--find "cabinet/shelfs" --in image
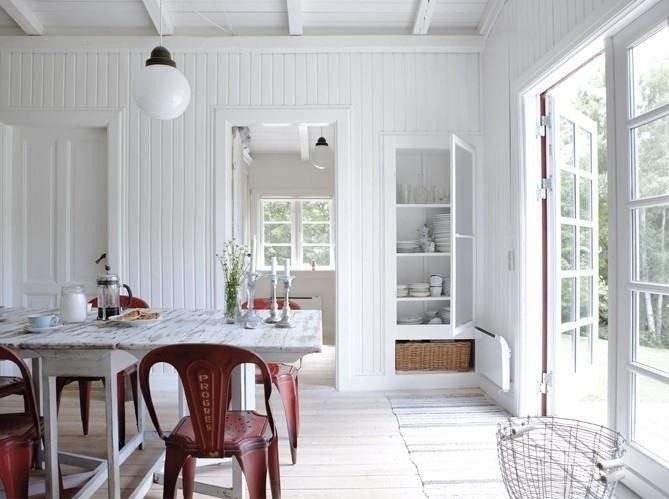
[383,135,511,395]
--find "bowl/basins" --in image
[440,305,451,323]
[424,309,440,324]
[429,275,443,297]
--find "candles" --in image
[250,235,290,277]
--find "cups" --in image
[86,303,92,313]
[398,182,412,204]
[432,184,450,204]
[27,313,59,328]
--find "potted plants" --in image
[214,237,251,322]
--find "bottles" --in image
[59,284,87,323]
[311,258,316,270]
[413,170,428,204]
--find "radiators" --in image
[475,326,511,394]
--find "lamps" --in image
[310,127,331,169]
[134,0,190,122]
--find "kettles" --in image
[95,272,132,322]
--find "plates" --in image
[428,317,442,324]
[108,313,164,327]
[431,213,451,252]
[25,326,58,334]
[397,284,408,297]
[408,282,431,297]
[397,315,423,324]
[396,240,418,253]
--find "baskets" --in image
[496,416,628,499]
[395,341,472,371]
